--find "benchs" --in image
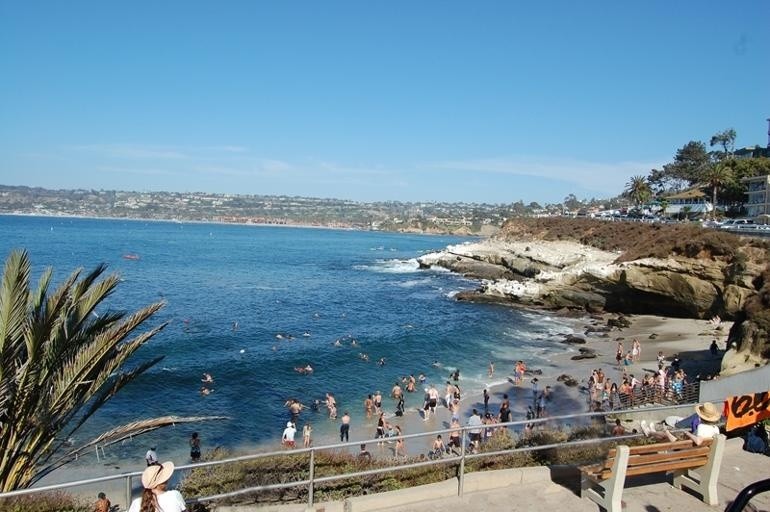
[576,432,727,512]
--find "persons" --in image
[710,341,719,358]
[127,460,188,512]
[612,419,624,436]
[588,340,689,423]
[189,433,201,462]
[640,402,722,451]
[467,360,553,454]
[695,367,721,382]
[201,319,462,463]
[93,491,112,512]
[144,443,158,466]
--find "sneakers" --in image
[640,420,651,438]
[650,422,656,434]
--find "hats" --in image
[141,461,175,489]
[151,444,158,448]
[695,401,721,423]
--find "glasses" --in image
[151,462,163,481]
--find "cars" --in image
[593,208,756,229]
